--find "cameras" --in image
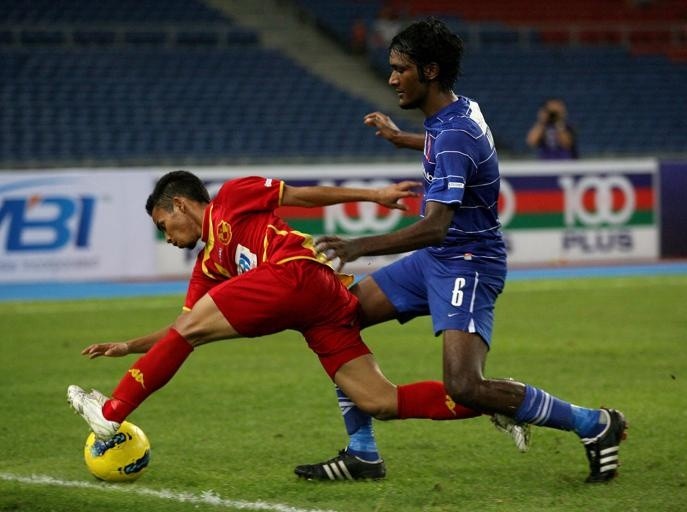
[547,111,558,127]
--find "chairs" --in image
[1,1,686,169]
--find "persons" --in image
[63,168,534,456]
[527,97,579,160]
[293,17,627,482]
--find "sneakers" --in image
[294,444,386,482]
[66,384,120,441]
[579,406,629,483]
[493,376,531,454]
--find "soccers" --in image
[84,420,151,483]
